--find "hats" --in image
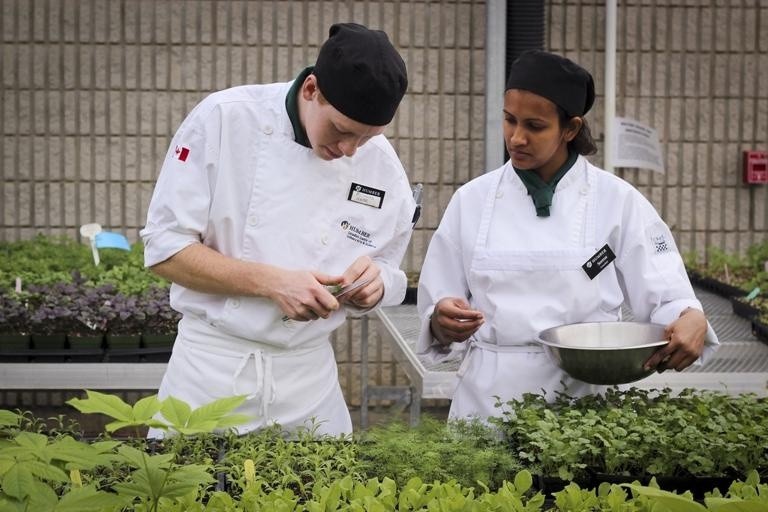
[502,48,596,117]
[310,23,408,127]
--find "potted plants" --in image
[684,238,768,345]
[0,266,184,364]
[57,433,500,507]
[514,388,768,502]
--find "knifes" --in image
[279,278,370,323]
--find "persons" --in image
[418,50,721,447]
[136,23,419,439]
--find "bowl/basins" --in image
[533,320,677,386]
